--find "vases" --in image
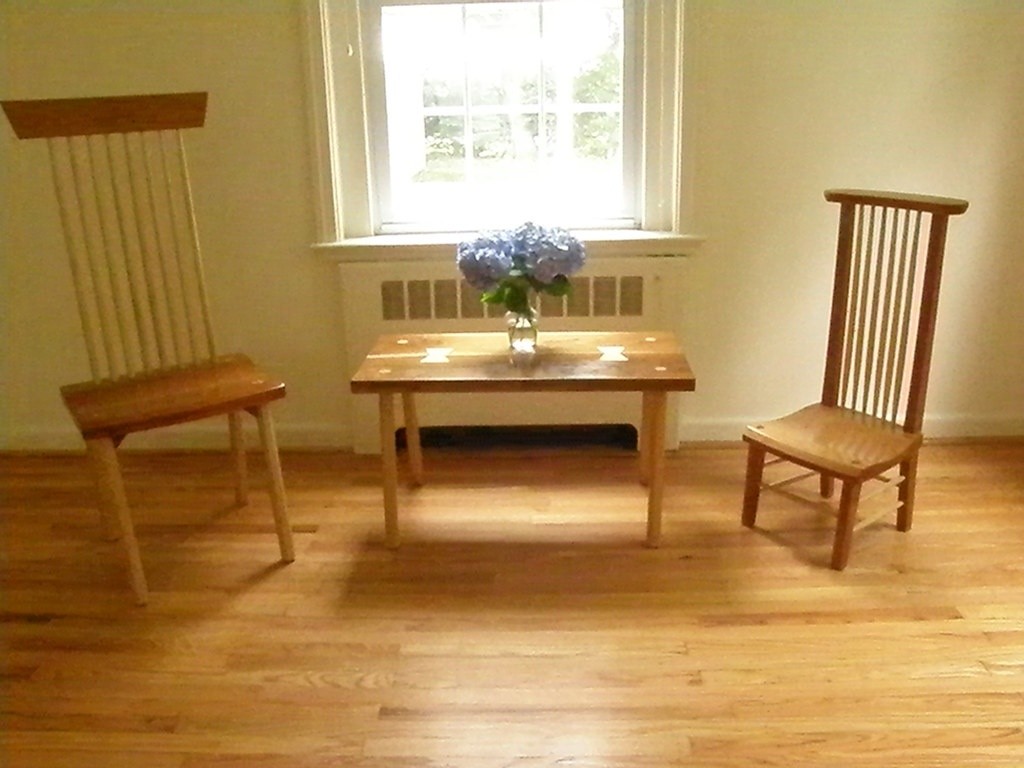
[504,310,537,351]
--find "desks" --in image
[350,332,697,549]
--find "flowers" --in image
[456,221,586,344]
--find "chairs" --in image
[0,92,295,607]
[742,190,969,571]
